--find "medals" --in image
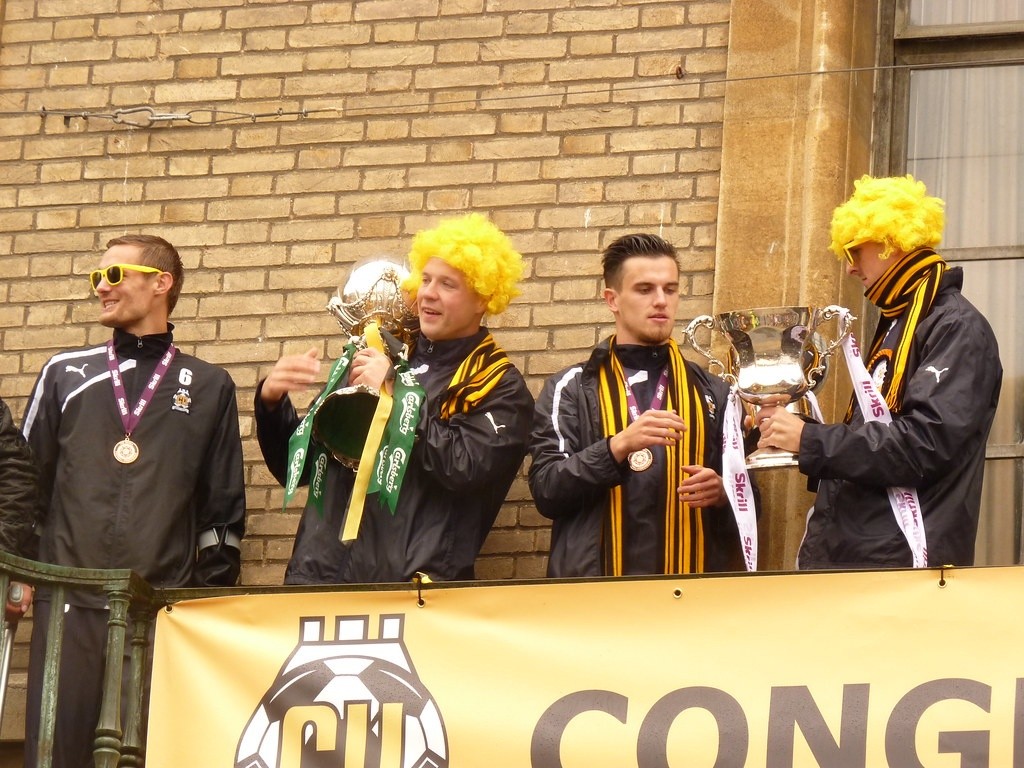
[623,444,654,471]
[111,431,141,464]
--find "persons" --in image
[527,234,761,578]
[253,211,536,585]
[755,172,1004,569]
[1,233,245,767]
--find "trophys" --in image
[322,252,419,405]
[681,303,859,470]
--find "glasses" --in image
[842,237,871,267]
[89,263,162,290]
